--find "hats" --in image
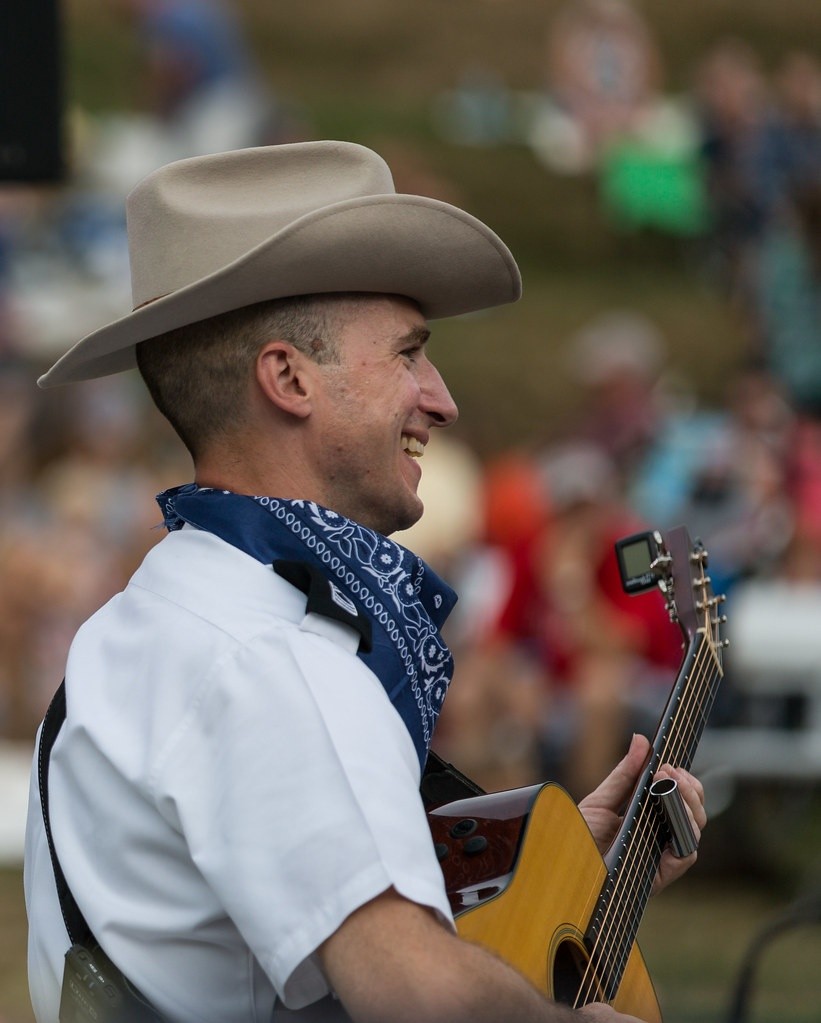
[29,140,523,383]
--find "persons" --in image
[22,140,706,1022]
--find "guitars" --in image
[415,523,736,1022]
